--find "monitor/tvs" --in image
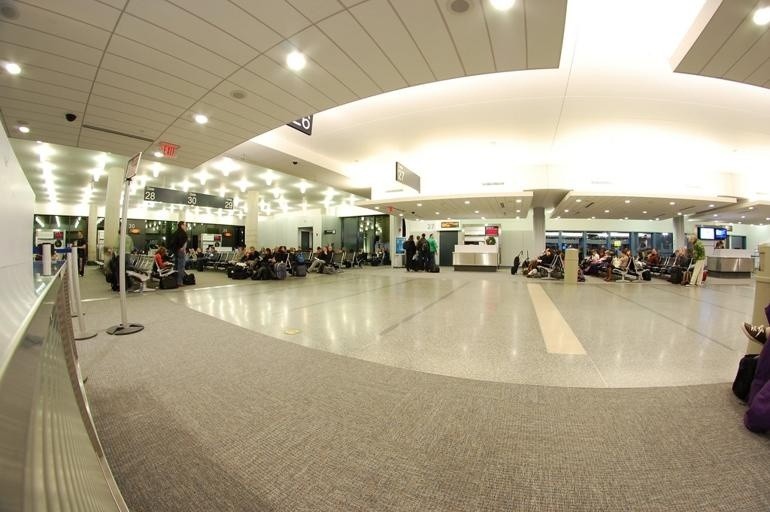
[484,226,498,236]
[715,228,728,240]
[699,227,715,240]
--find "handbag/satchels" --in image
[733,354,760,403]
[613,257,622,269]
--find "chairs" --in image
[95,250,366,292]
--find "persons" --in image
[742,302,769,344]
[524,233,705,288]
[405,233,438,272]
[170,221,188,286]
[714,240,724,248]
[104,228,135,292]
[227,245,304,277]
[306,244,335,273]
[186,244,219,271]
[129,247,170,269]
[376,244,388,266]
[76,230,88,278]
[146,240,165,249]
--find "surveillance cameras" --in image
[65,114,77,122]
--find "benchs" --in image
[533,255,697,284]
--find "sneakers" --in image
[741,321,767,345]
